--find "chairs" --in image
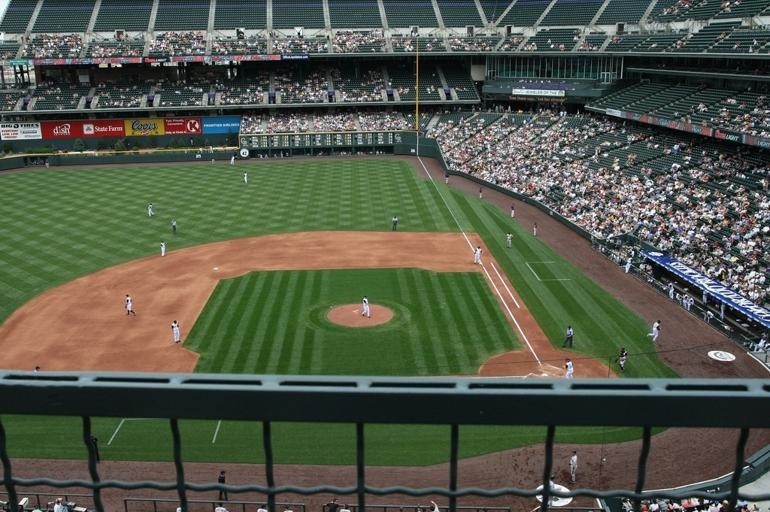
[620,495,762,512]
[0,0,436,157]
[427,3,768,313]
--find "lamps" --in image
[620,495,762,512]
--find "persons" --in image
[244,171,247,183]
[33,498,759,512]
[2,29,436,169]
[533,222,537,236]
[562,325,574,348]
[647,320,662,341]
[160,241,167,256]
[171,218,177,231]
[218,471,228,501]
[474,246,483,264]
[171,320,181,343]
[148,203,154,216]
[362,296,371,317]
[437,0,770,366]
[90,435,100,462]
[565,358,574,379]
[547,474,555,509]
[511,203,515,217]
[569,450,578,482]
[615,348,627,370]
[392,216,398,231]
[506,232,513,248]
[34,366,40,372]
[445,172,449,184]
[479,187,482,198]
[125,294,136,316]
